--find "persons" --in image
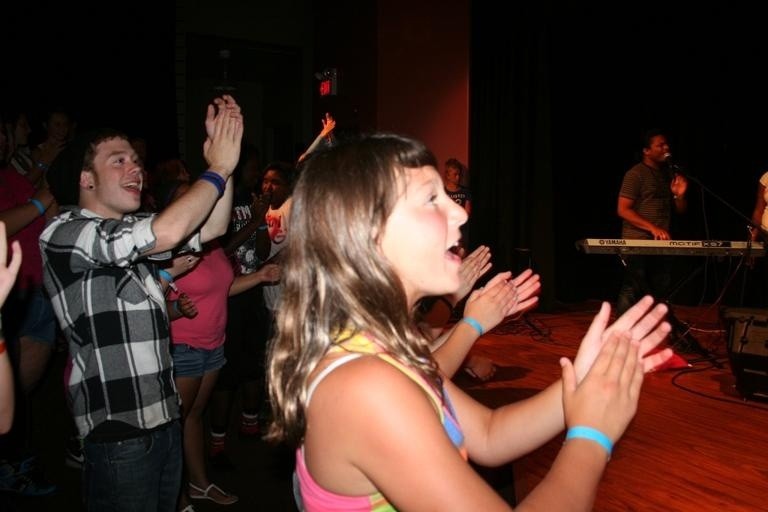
[615,132,688,313]
[0,96,541,511]
[749,172,768,309]
[269,131,673,510]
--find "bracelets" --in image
[566,426,612,461]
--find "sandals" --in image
[180,505,194,512]
[189,482,238,504]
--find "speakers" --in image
[719,306,767,402]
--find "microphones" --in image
[663,152,676,183]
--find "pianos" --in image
[572,238,764,256]
